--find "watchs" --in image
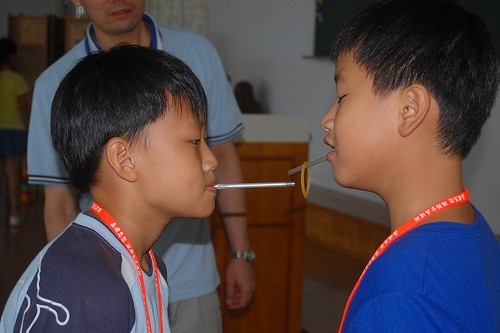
[231,250,257,262]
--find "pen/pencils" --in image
[213,181,296,189]
[287,156,327,175]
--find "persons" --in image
[27,0,256,333]
[321,0,500,333]
[0,44,218,333]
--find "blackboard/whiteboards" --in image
[315,0,500,62]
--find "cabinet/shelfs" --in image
[207,143,309,333]
